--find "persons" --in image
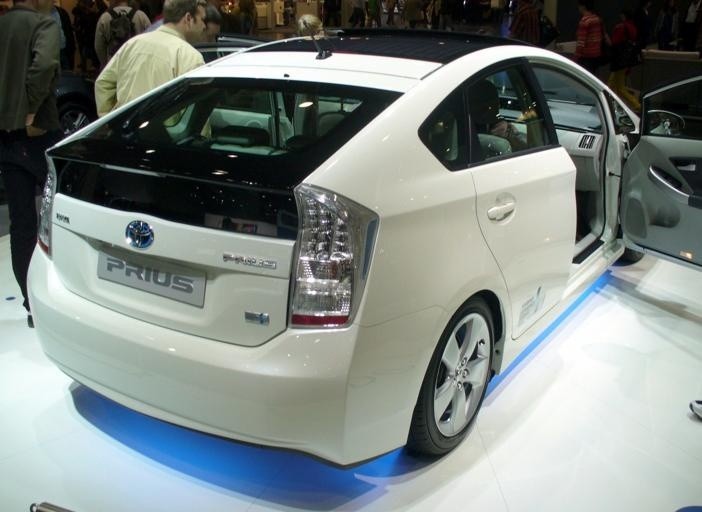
[321,1,700,106]
[1,1,66,331]
[469,80,528,147]
[63,1,258,135]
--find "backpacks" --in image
[104,6,138,57]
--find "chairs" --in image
[479,133,513,157]
[209,113,351,157]
